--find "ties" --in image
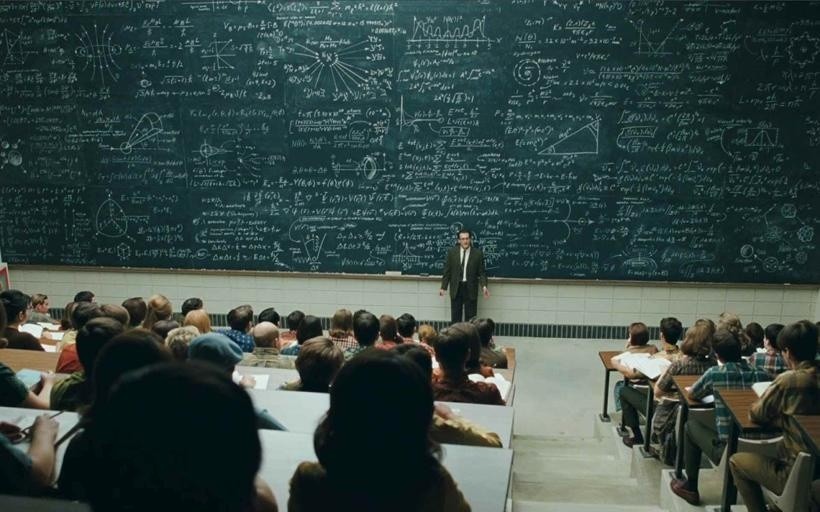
[461,249,466,281]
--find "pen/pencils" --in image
[26,410,65,430]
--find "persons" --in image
[438,229,490,324]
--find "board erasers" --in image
[384,271,402,276]
[420,273,430,276]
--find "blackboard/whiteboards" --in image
[0,0,820,289]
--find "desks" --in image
[1,321,88,478]
[230,327,516,449]
[254,428,517,512]
[597,350,820,511]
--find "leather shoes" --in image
[670,477,700,505]
[621,436,634,449]
